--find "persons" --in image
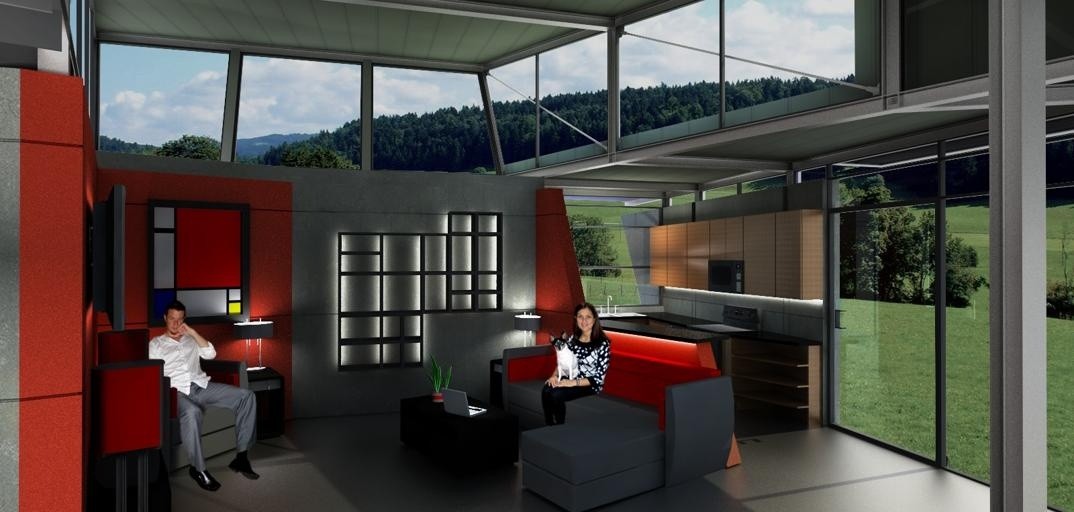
[542,303,610,426]
[148,300,259,491]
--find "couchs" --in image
[503,343,738,511]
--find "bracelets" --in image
[576,379,579,386]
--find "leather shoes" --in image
[189,464,221,491]
[228,457,260,480]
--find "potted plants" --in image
[424,355,454,402]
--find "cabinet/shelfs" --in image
[648,208,824,305]
[733,338,823,430]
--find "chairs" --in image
[152,359,250,477]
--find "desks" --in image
[247,370,285,441]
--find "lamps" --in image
[512,312,544,348]
[234,320,274,374]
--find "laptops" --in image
[441,386,489,418]
